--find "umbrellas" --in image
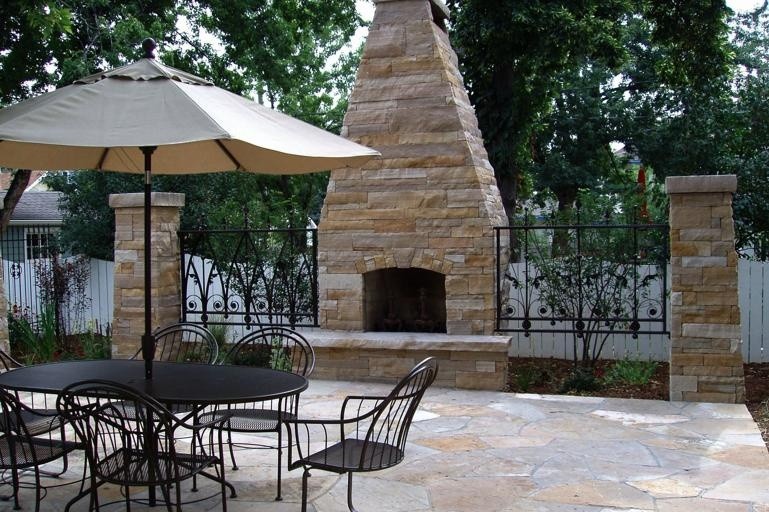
[1,38,383,507]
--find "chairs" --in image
[191,327,317,499]
[57,380,235,512]
[282,355,437,510]
[1,358,310,498]
[101,321,219,491]
[0,350,98,511]
